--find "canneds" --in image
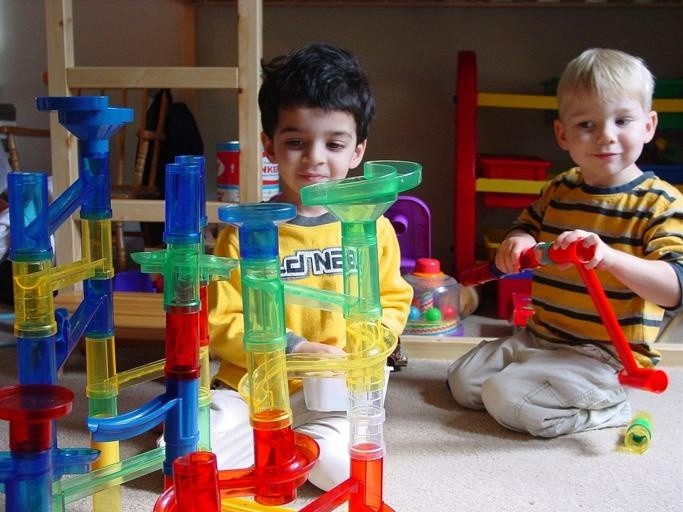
[214,139,281,204]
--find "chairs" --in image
[0,64,174,271]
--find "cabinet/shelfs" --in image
[44,0,264,356]
[455,48,682,293]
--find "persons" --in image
[155,42,415,494]
[444,47,683,442]
